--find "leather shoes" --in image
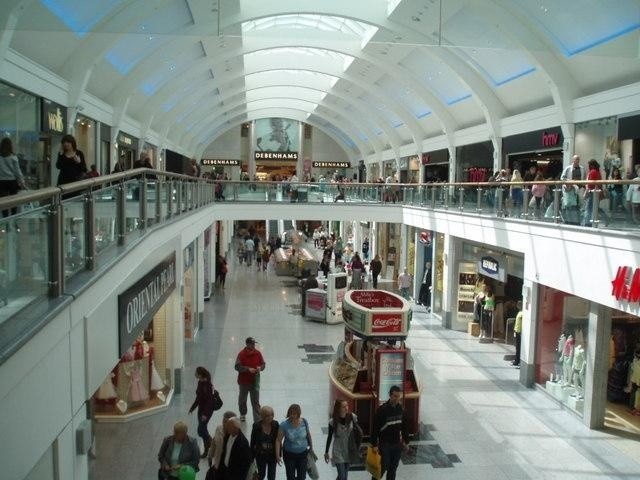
[206,437,212,447]
[202,448,209,457]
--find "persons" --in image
[551,329,588,399]
[1,134,200,235]
[202,169,402,204]
[417,262,431,304]
[471,276,496,341]
[512,301,523,368]
[219,222,411,300]
[157,336,413,480]
[408,155,640,229]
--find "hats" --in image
[246,338,257,344]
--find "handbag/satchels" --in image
[247,462,259,480]
[349,431,363,464]
[158,461,169,480]
[211,386,224,410]
[365,446,381,479]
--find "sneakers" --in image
[240,415,245,421]
